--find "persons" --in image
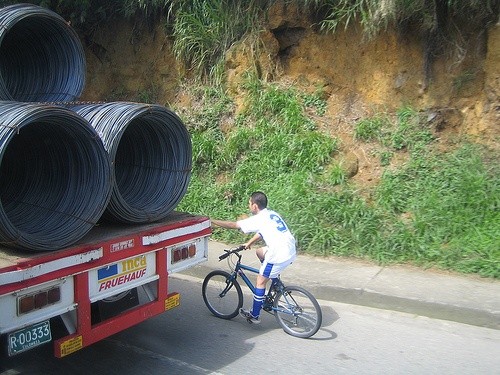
[210,191,298,325]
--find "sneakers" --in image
[239,308,261,324]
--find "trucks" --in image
[0,215,210,356]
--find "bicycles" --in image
[201,245,323,338]
